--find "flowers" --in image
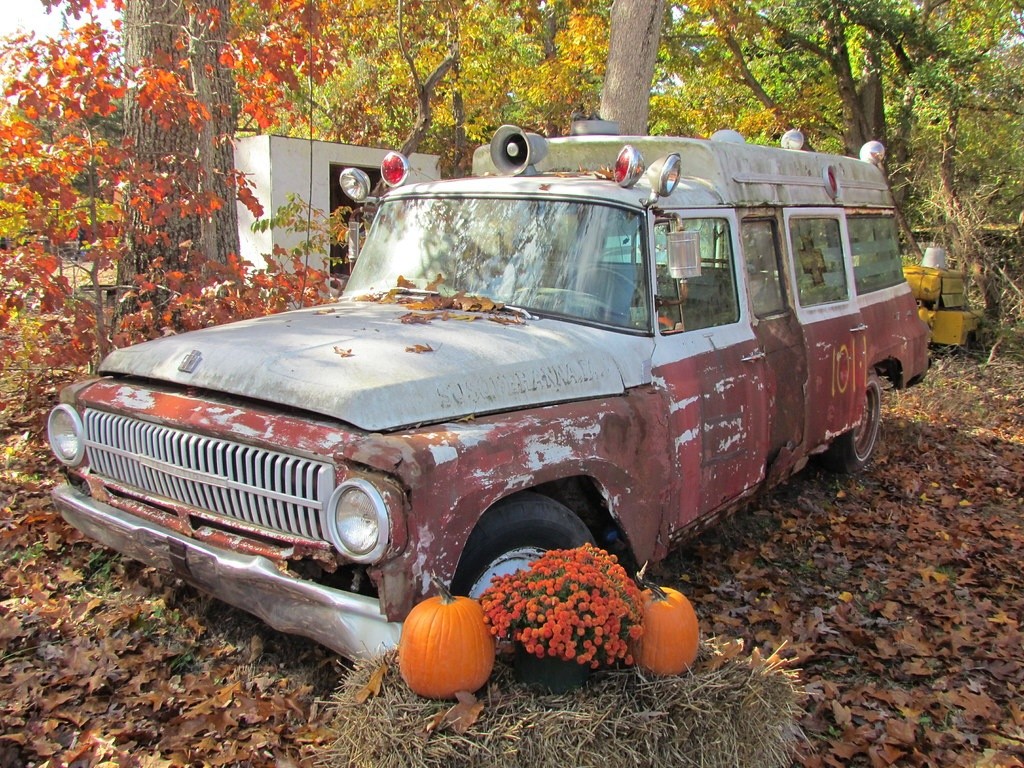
[477,541,647,671]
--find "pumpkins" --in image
[397,576,495,700]
[633,560,699,674]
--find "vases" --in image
[507,630,598,692]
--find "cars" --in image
[49,117,930,664]
